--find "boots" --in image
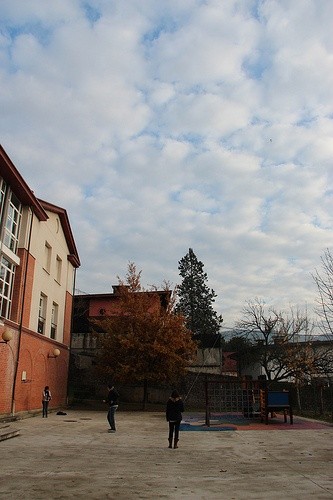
[168,437,172,448]
[174,438,179,449]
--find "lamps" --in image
[47,349,60,358]
[0,330,13,344]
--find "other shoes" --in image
[108,429,116,432]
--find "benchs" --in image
[267,391,293,424]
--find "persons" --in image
[165,392,185,448]
[103,383,119,431]
[42,386,51,418]
